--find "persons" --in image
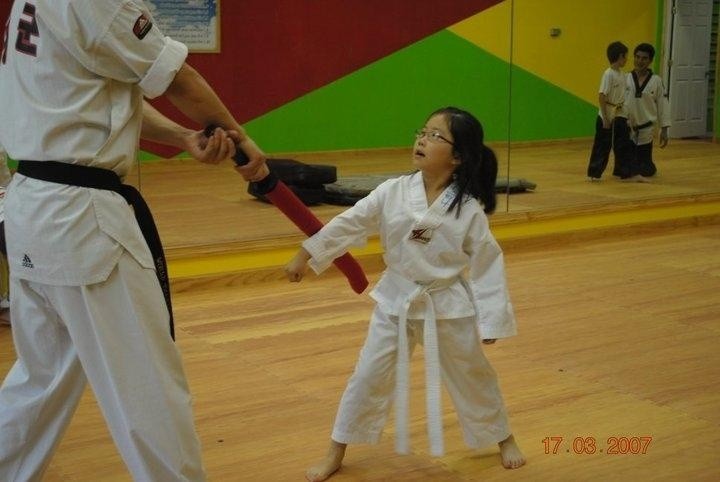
[0,138,13,327]
[627,43,671,177]
[588,41,650,183]
[286,106,527,481]
[0,0,269,480]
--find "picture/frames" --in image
[143,0,222,54]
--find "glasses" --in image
[415,127,453,146]
